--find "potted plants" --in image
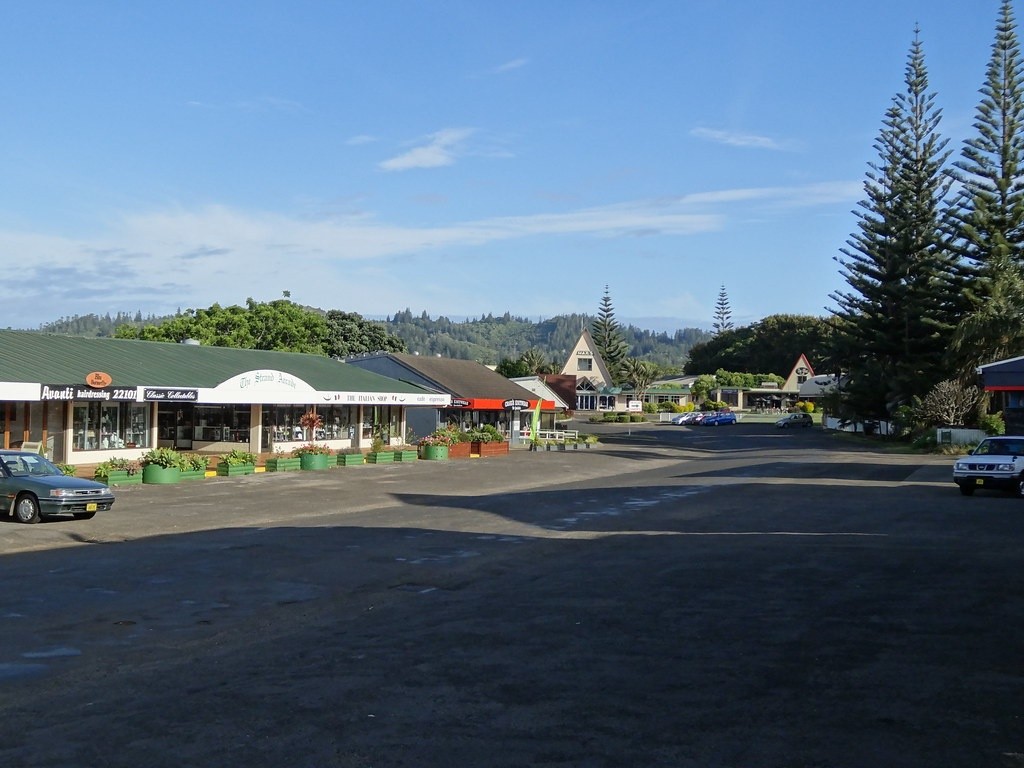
[433,422,509,459]
[93,440,419,488]
[530,436,600,453]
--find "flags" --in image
[529,399,542,439]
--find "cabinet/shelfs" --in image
[72,401,151,451]
[176,404,250,447]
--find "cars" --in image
[776,413,814,428]
[671,411,737,427]
[953,436,1024,499]
[0,450,116,524]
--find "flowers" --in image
[302,444,334,455]
[420,434,450,447]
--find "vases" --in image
[424,446,447,460]
[300,454,328,470]
